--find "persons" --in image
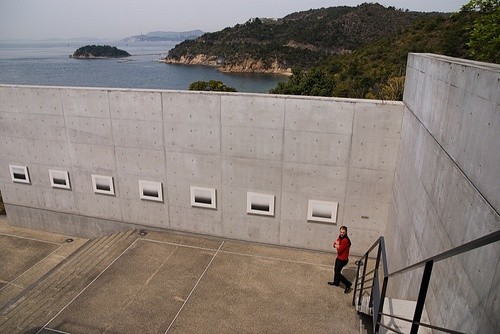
[327,226,353,294]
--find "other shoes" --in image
[328,282,340,286]
[344,283,352,293]
